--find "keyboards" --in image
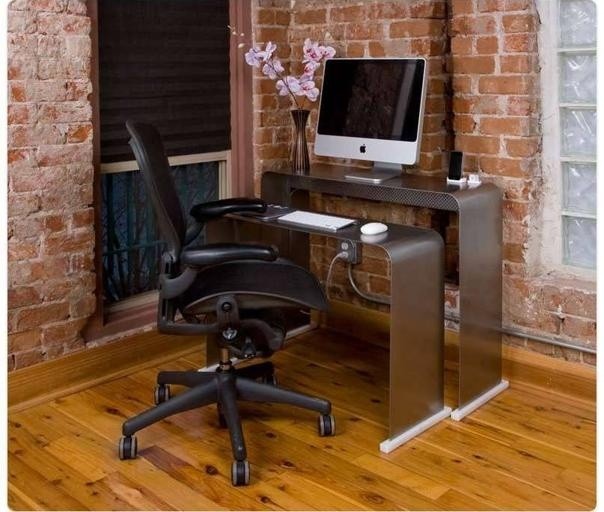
[277,210,356,232]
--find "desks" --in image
[258,165,508,424]
[213,202,455,453]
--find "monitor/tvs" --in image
[314,58,427,182]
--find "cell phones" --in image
[449,151,463,180]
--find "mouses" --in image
[360,223,388,233]
[361,232,389,244]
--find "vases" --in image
[290,111,309,173]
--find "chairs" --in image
[117,118,335,485]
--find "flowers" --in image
[227,23,337,110]
